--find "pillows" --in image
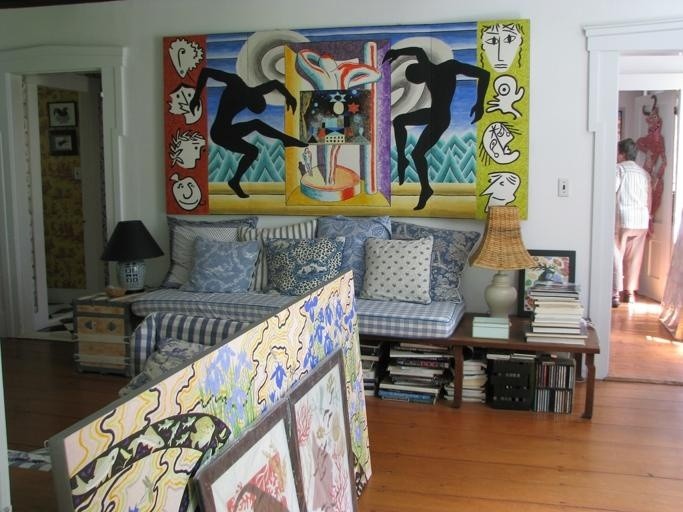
[168,216,256,291]
[390,223,478,304]
[123,340,215,394]
[266,237,344,299]
[239,218,317,292]
[364,237,433,304]
[318,216,392,297]
[179,239,259,296]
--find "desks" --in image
[447,314,598,421]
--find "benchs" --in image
[132,285,467,339]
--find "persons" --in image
[612,138,653,308]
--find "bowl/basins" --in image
[105,288,127,296]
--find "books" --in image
[377,342,455,405]
[486,352,510,361]
[462,359,488,404]
[360,341,377,396]
[525,284,589,346]
[535,353,575,413]
[510,351,536,359]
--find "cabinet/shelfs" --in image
[72,290,150,377]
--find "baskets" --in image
[488,358,536,411]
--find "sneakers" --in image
[624,294,636,303]
[612,295,621,307]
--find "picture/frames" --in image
[518,250,577,317]
[189,398,311,512]
[48,131,78,156]
[47,102,76,126]
[287,344,358,512]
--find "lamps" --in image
[100,220,164,294]
[469,207,540,326]
